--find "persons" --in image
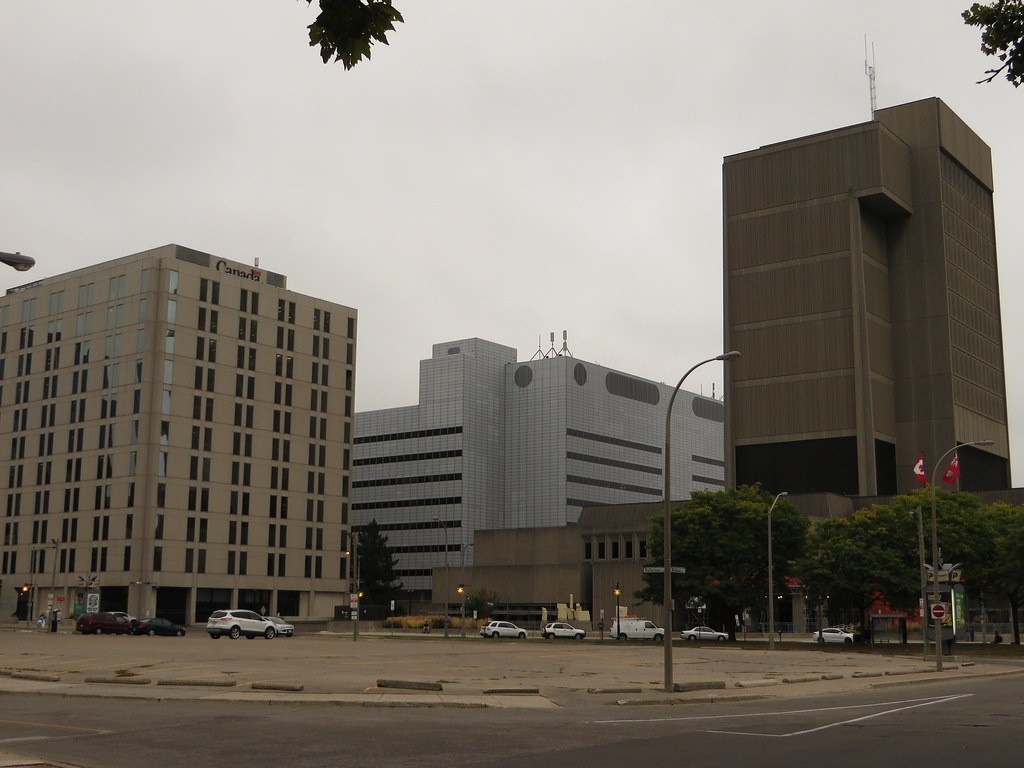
[990,630,998,644]
[422,621,430,633]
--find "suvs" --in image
[541,622,586,641]
[206,609,295,640]
[75,611,137,635]
[480,621,528,639]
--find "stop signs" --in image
[930,603,945,619]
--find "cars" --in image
[132,617,186,637]
[813,627,854,644]
[679,626,728,641]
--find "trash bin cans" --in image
[942,638,956,655]
[853,633,862,642]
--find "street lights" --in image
[458,584,466,638]
[407,588,414,615]
[929,440,995,672]
[346,551,360,636]
[767,491,788,649]
[613,581,622,640]
[664,351,743,693]
[432,516,449,639]
[460,543,474,640]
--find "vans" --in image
[609,617,664,641]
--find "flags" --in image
[913,454,928,485]
[943,453,960,485]
